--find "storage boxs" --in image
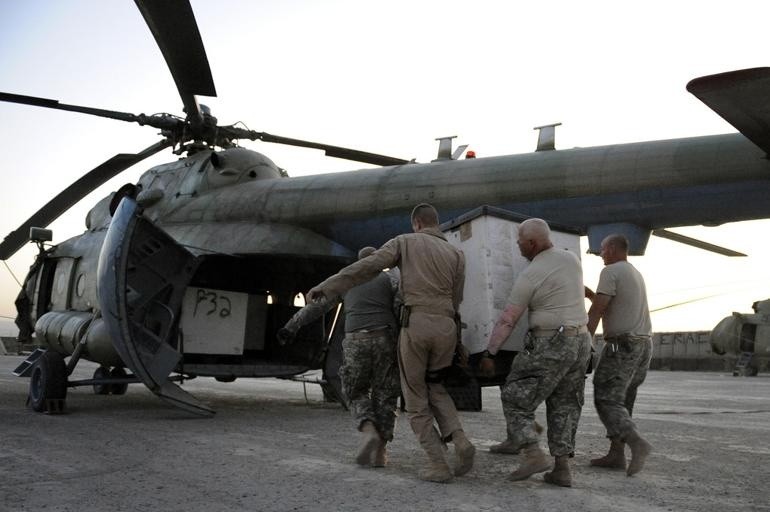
[385,206,596,389]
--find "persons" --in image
[307,202,476,485]
[275,246,400,469]
[488,384,543,456]
[581,232,658,477]
[478,218,593,487]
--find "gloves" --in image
[274,328,297,346]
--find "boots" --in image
[544,453,572,487]
[589,438,626,470]
[376,437,389,467]
[417,442,452,483]
[489,435,521,455]
[355,419,381,466]
[624,428,654,477]
[451,430,475,477]
[504,441,551,482]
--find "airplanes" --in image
[710,300,769,376]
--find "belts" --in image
[344,329,387,340]
[532,324,589,338]
[409,305,456,318]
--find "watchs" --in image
[484,350,498,361]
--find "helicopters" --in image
[2,1,770,412]
